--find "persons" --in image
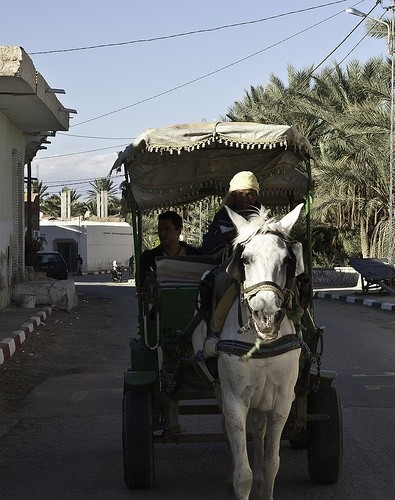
[140,209,200,283]
[200,170,261,259]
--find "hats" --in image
[228,171,259,195]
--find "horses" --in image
[192,202,304,500]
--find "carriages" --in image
[121,119,342,499]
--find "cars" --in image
[28,250,68,281]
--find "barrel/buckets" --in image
[20,294,36,309]
